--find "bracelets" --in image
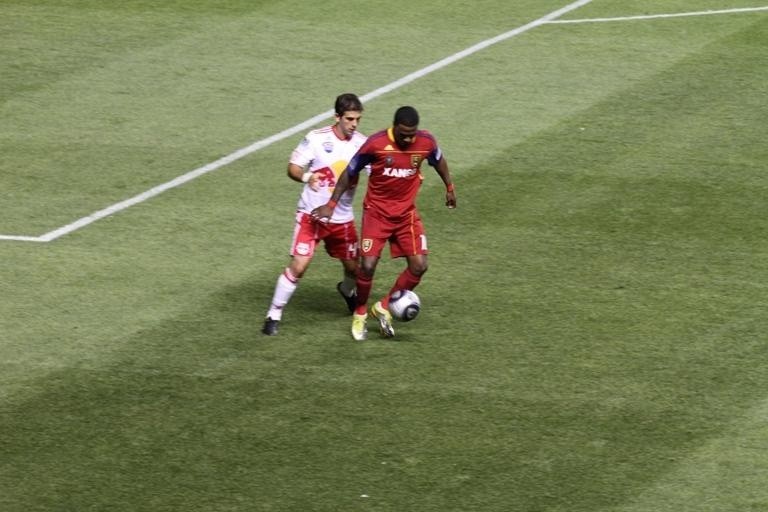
[446,183,454,192]
[301,172,311,183]
[327,200,336,209]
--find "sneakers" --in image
[369,301,395,338]
[263,308,283,336]
[336,281,357,313]
[351,311,368,341]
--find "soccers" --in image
[387,288,420,321]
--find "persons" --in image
[261,93,372,336]
[308,105,456,341]
